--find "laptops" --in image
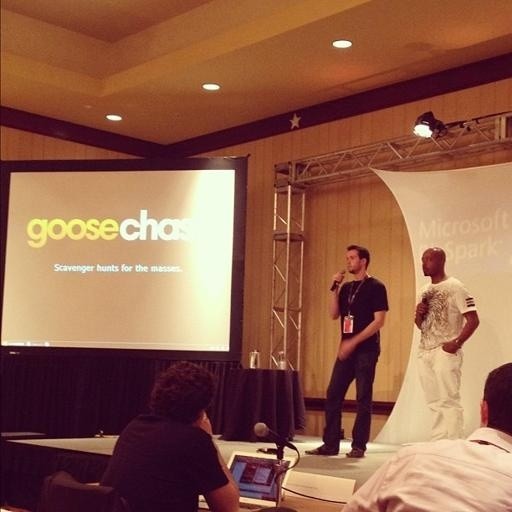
[199,451,295,508]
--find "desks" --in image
[221,365,310,446]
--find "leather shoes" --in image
[346,449,364,457]
[305,448,338,455]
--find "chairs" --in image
[35,469,132,512]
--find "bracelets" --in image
[454,337,464,349]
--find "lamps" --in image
[410,109,449,141]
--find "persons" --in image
[340,363,511,512]
[306,244,388,456]
[415,248,479,441]
[99,362,243,512]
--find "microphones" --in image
[330,269,346,292]
[422,293,427,320]
[254,422,296,450]
